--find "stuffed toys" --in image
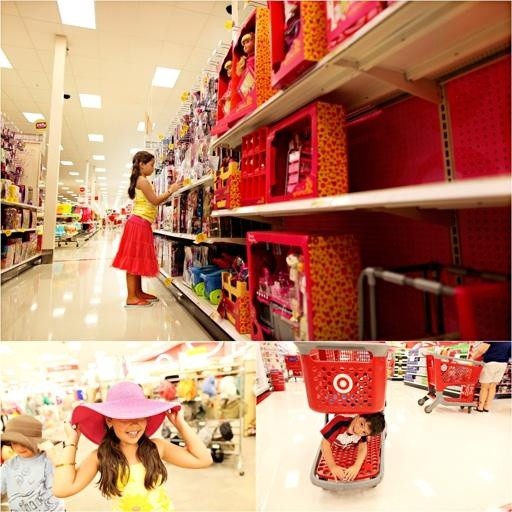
[183,0,382,341]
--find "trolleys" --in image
[418,352,486,413]
[293,341,390,491]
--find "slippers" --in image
[124,293,157,309]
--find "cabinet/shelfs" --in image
[255,340,510,397]
[0,0,512,340]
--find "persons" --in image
[52,382,213,512]
[0,414,67,512]
[111,151,182,308]
[183,0,382,341]
[320,411,386,482]
[470,342,511,412]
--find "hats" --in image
[0,414,47,453]
[71,383,183,446]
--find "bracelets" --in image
[54,463,77,468]
[63,444,78,450]
[168,190,172,196]
